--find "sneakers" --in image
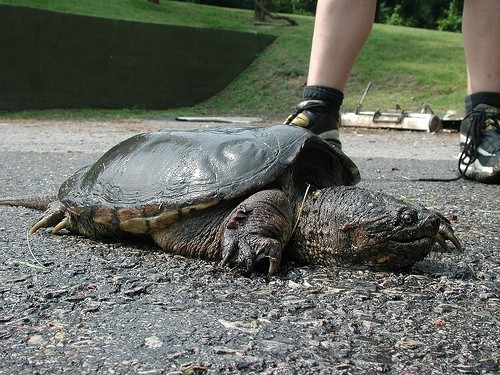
[282,100,342,151]
[458,103,500,184]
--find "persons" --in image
[283,0,500,181]
